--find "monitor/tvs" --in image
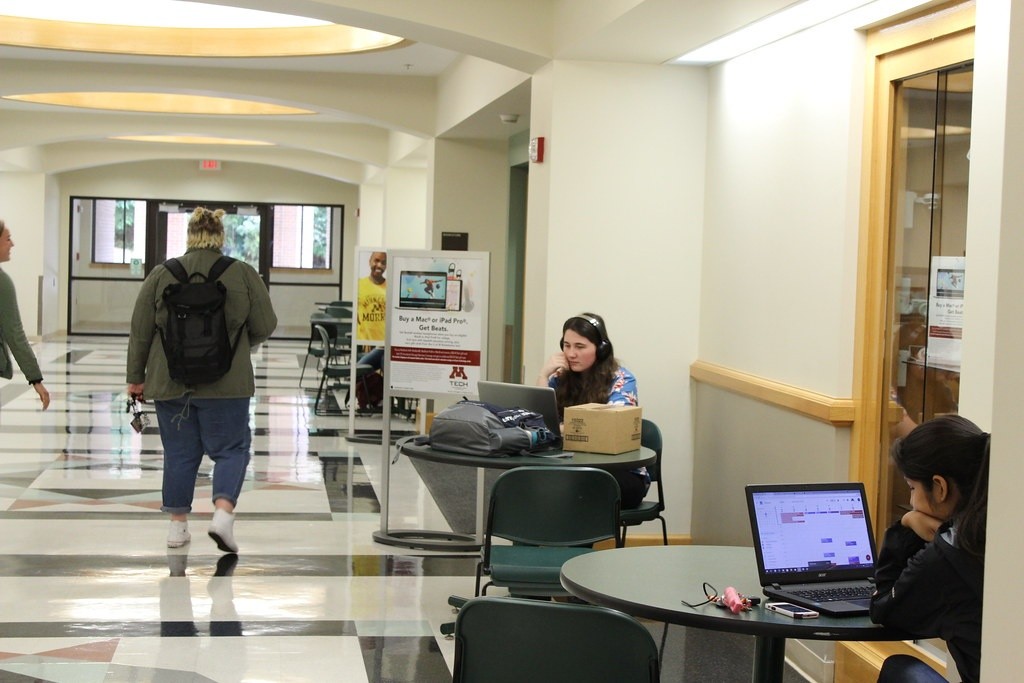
[399,271,447,309]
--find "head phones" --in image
[559,315,612,363]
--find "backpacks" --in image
[427,397,563,457]
[156,256,247,386]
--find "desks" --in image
[310,316,354,410]
[391,431,656,636]
[560,543,940,682]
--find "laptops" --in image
[477,380,563,439]
[746,482,893,618]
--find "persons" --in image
[357,346,384,408]
[355,252,386,340]
[869,414,991,683]
[0,220,50,412]
[889,323,960,530]
[126,206,278,553]
[421,279,443,297]
[511,313,651,606]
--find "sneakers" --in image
[207,508,238,552]
[208,554,238,601]
[167,518,190,548]
[168,548,188,572]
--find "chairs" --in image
[299,287,423,416]
[473,462,616,602]
[609,421,672,548]
[451,598,662,683]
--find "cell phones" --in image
[765,602,819,619]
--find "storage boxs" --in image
[563,401,642,456]
